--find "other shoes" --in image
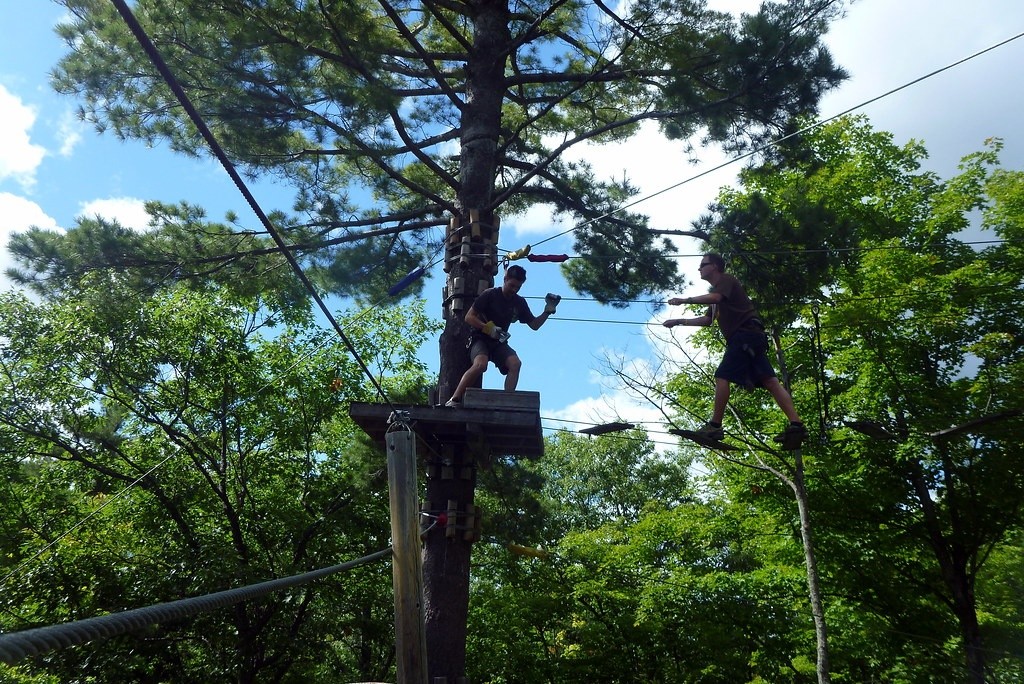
[692,424,725,440]
[773,424,809,442]
[445,399,460,408]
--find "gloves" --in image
[481,321,501,339]
[544,293,561,314]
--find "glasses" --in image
[700,263,712,268]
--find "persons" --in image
[443,265,561,410]
[660,252,811,446]
